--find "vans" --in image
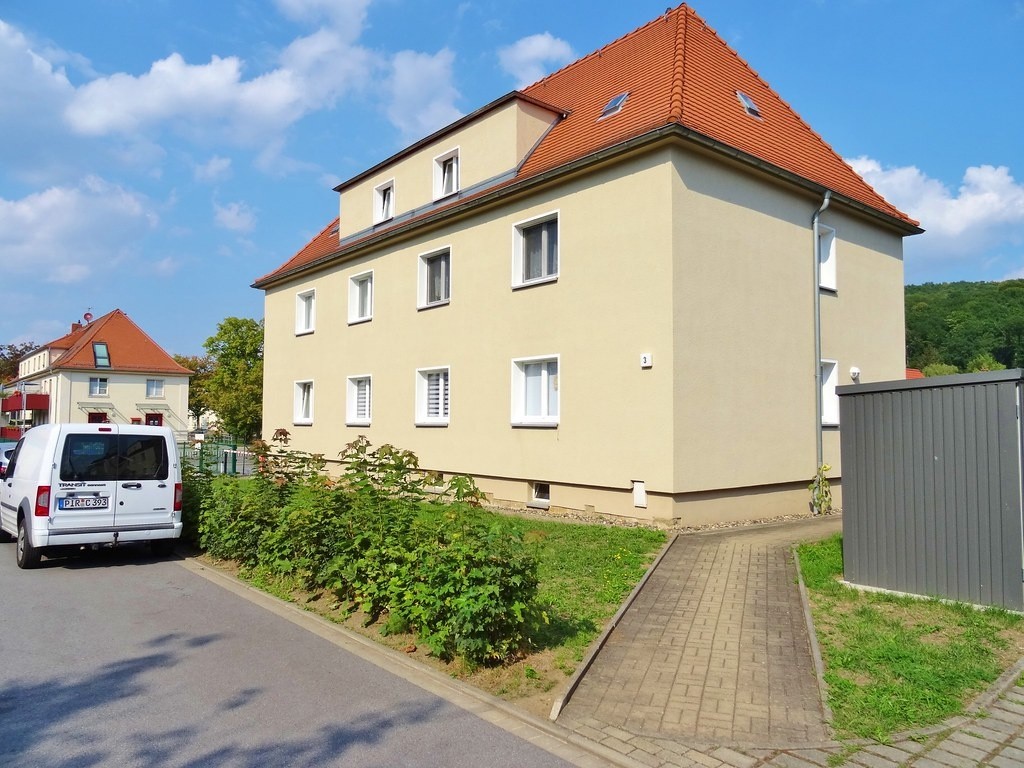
[1,421,183,568]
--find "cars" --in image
[0,441,18,480]
